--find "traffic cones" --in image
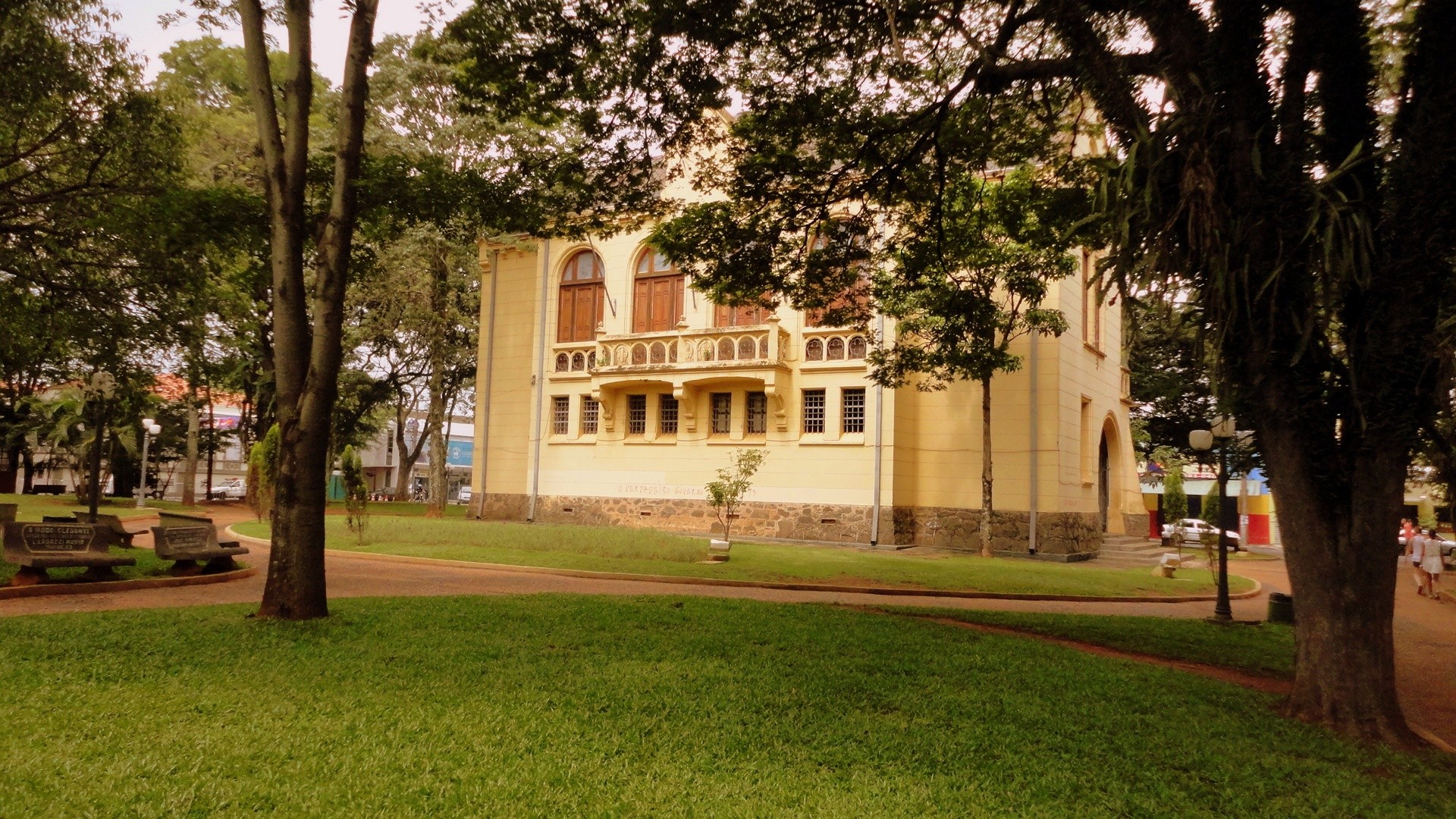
[385,495,388,501]
[371,494,375,500]
[378,495,382,501]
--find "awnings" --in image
[1140,479,1262,496]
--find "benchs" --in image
[207,491,225,500]
[151,525,249,575]
[159,511,241,548]
[72,511,149,549]
[71,485,105,494]
[0,503,18,522]
[28,485,67,495]
[43,516,77,522]
[0,522,137,587]
[144,491,164,500]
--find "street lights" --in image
[135,418,162,509]
[1190,416,1236,623]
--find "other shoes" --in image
[1418,586,1423,595]
[1435,595,1440,601]
[1428,595,1433,599]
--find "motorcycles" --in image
[415,490,426,504]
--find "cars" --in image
[1398,528,1456,556]
[1159,518,1241,546]
[210,479,247,499]
[375,486,395,499]
[458,486,471,504]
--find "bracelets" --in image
[1419,565,1422,567]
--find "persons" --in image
[1419,529,1445,599]
[1400,516,1419,555]
[414,484,426,503]
[1404,526,1427,595]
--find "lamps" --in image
[708,538,732,552]
[1161,553,1180,565]
